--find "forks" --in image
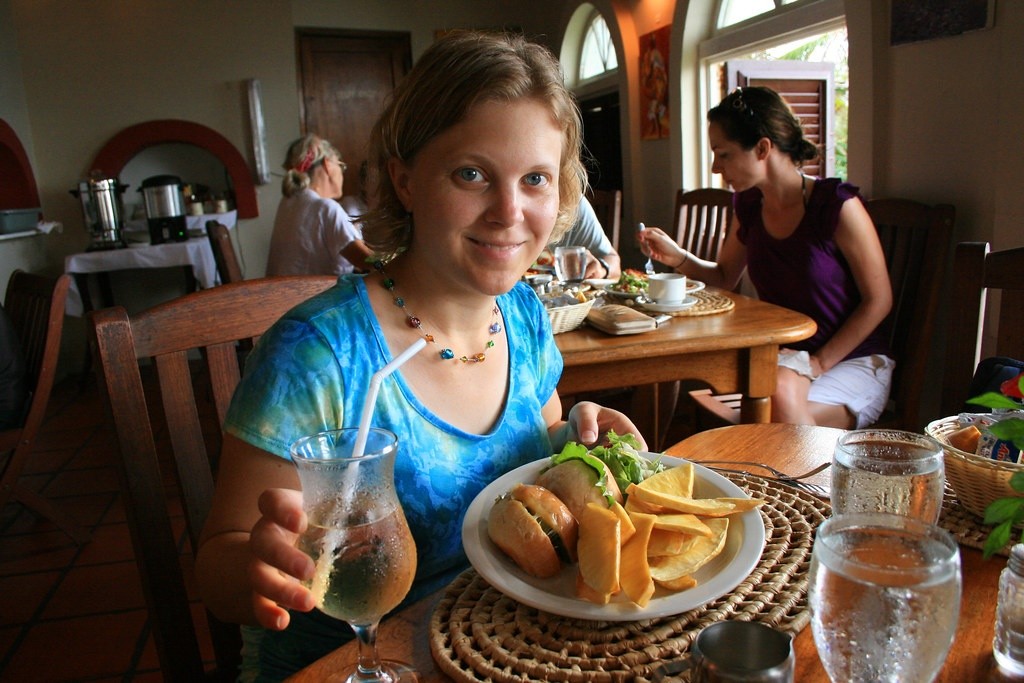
[639,222,656,274]
[691,459,830,481]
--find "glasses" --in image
[728,86,774,143]
[327,159,347,175]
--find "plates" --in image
[636,294,698,311]
[461,450,766,621]
[604,279,705,298]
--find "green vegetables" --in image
[539,429,666,506]
[608,270,651,293]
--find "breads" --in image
[488,454,629,578]
[947,424,983,452]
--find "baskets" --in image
[538,292,596,335]
[924,413,1024,531]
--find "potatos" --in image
[576,464,766,607]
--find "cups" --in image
[806,511,962,683]
[827,429,945,528]
[692,621,796,683]
[647,273,687,304]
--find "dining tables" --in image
[65,210,238,371]
[555,285,818,424]
[282,424,1024,683]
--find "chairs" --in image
[688,196,956,433]
[89,274,338,683]
[668,188,733,279]
[0,268,91,547]
[939,241,1024,418]
[589,189,622,251]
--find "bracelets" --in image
[673,249,690,269]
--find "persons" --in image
[266,134,397,278]
[193,28,650,683]
[545,184,622,280]
[635,85,895,430]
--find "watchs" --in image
[597,257,610,279]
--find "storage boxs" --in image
[0,207,45,233]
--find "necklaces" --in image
[800,173,808,214]
[373,257,503,364]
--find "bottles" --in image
[991,542,1024,676]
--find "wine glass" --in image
[290,427,423,683]
[553,245,587,291]
